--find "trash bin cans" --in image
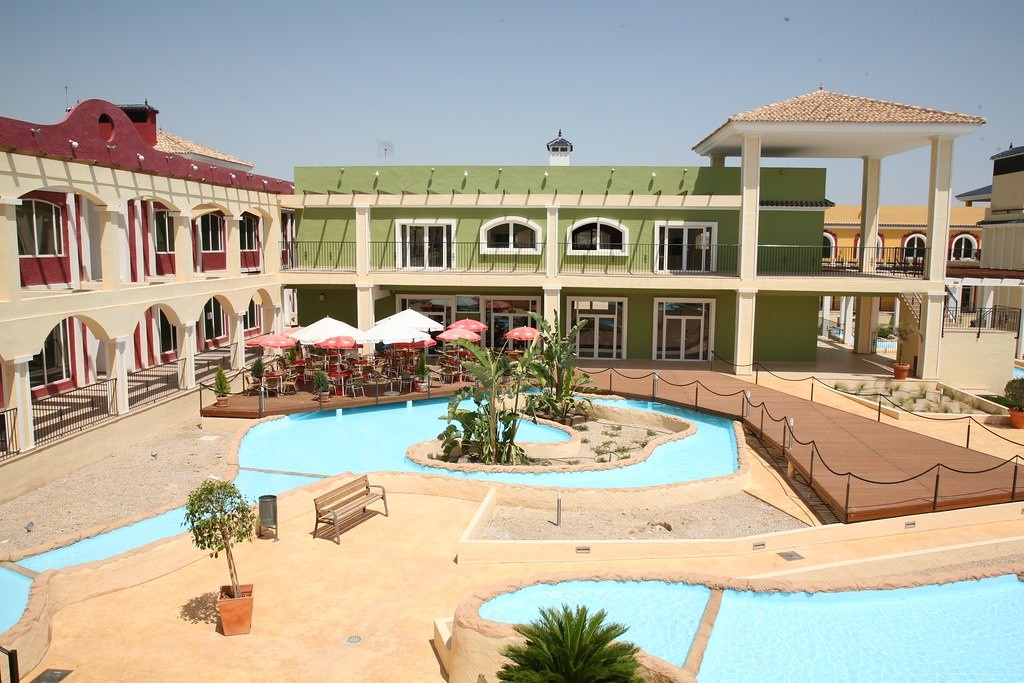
[0,414,7,451]
[258,494,279,542]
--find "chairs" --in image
[245,349,526,400]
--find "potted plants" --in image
[499,355,513,384]
[313,371,332,403]
[213,362,232,408]
[886,320,923,380]
[181,478,262,636]
[415,354,430,392]
[1003,376,1024,429]
[250,356,265,383]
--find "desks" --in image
[261,371,286,395]
[394,349,419,352]
[290,360,308,367]
[328,369,353,397]
[304,368,322,384]
[323,351,341,356]
[505,350,524,354]
[312,360,329,369]
[355,363,372,367]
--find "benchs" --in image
[311,474,390,544]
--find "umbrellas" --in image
[447,317,489,333]
[356,318,431,391]
[504,326,543,351]
[288,315,365,373]
[313,336,364,384]
[431,298,512,312]
[436,328,481,359]
[280,324,306,355]
[247,334,295,359]
[375,306,445,367]
[392,338,437,375]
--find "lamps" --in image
[320,293,325,301]
[68,140,656,191]
